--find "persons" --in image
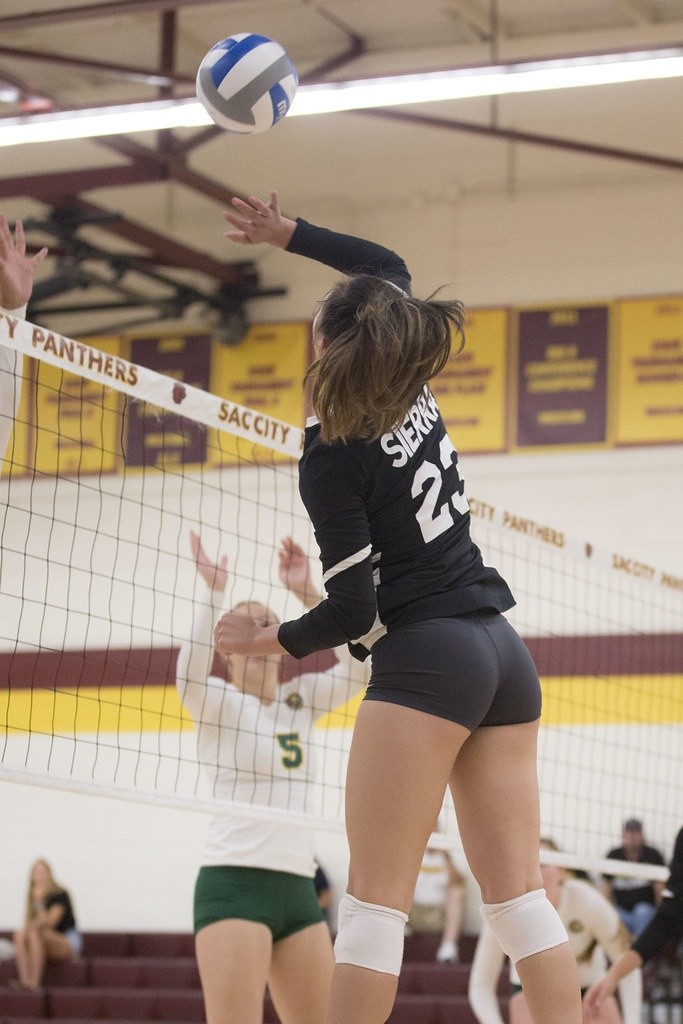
[211,189,589,1024]
[176,531,371,1024]
[313,816,644,1024]
[12,855,85,994]
[585,824,683,1018]
[599,811,672,939]
[0,212,48,464]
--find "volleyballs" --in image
[197,34,299,135]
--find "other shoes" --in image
[437,945,460,963]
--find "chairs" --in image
[0,929,511,1024]
